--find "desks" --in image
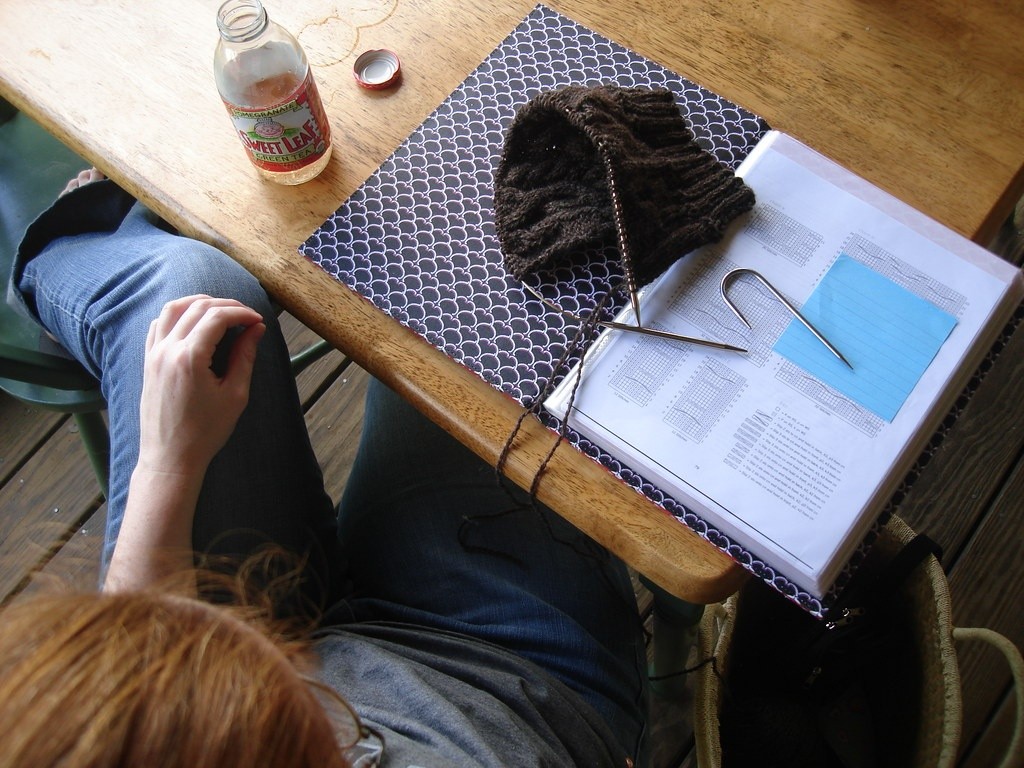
[2,0,1022,768]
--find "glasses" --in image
[293,676,389,767]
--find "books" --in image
[295,2,1024,599]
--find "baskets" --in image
[691,514,1024,768]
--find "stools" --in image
[0,99,362,505]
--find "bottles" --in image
[213,0,332,185]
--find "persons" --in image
[0,163,649,768]
